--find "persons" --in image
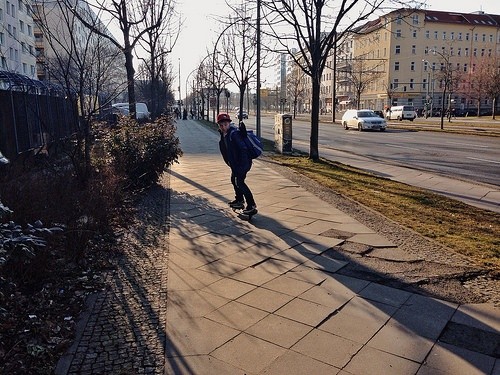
[422,109,430,118]
[182,108,186,120]
[384,108,392,121]
[189,108,194,119]
[174,108,180,119]
[216,114,264,213]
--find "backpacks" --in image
[238,121,263,159]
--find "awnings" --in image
[339,101,352,106]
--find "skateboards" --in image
[227,200,258,220]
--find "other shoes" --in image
[228,199,244,205]
[243,205,257,212]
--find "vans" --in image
[385,105,416,122]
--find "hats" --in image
[217,114,232,123]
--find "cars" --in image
[234,110,249,119]
[341,109,387,132]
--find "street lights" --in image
[197,51,221,121]
[185,66,203,116]
[429,49,453,122]
